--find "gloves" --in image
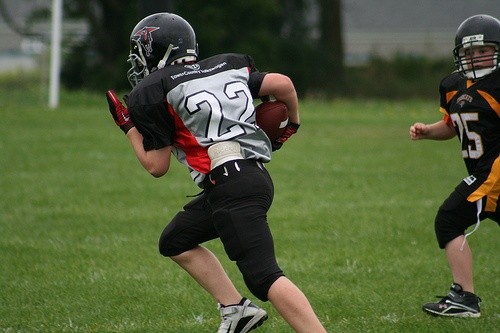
[106,88,136,135]
[271,121,300,152]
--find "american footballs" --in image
[254,100,290,142]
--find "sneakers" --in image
[423,283,482,318]
[217,297,269,333]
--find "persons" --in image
[106,13,329,333]
[410,15,500,318]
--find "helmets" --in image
[126,13,199,78]
[453,14,500,80]
